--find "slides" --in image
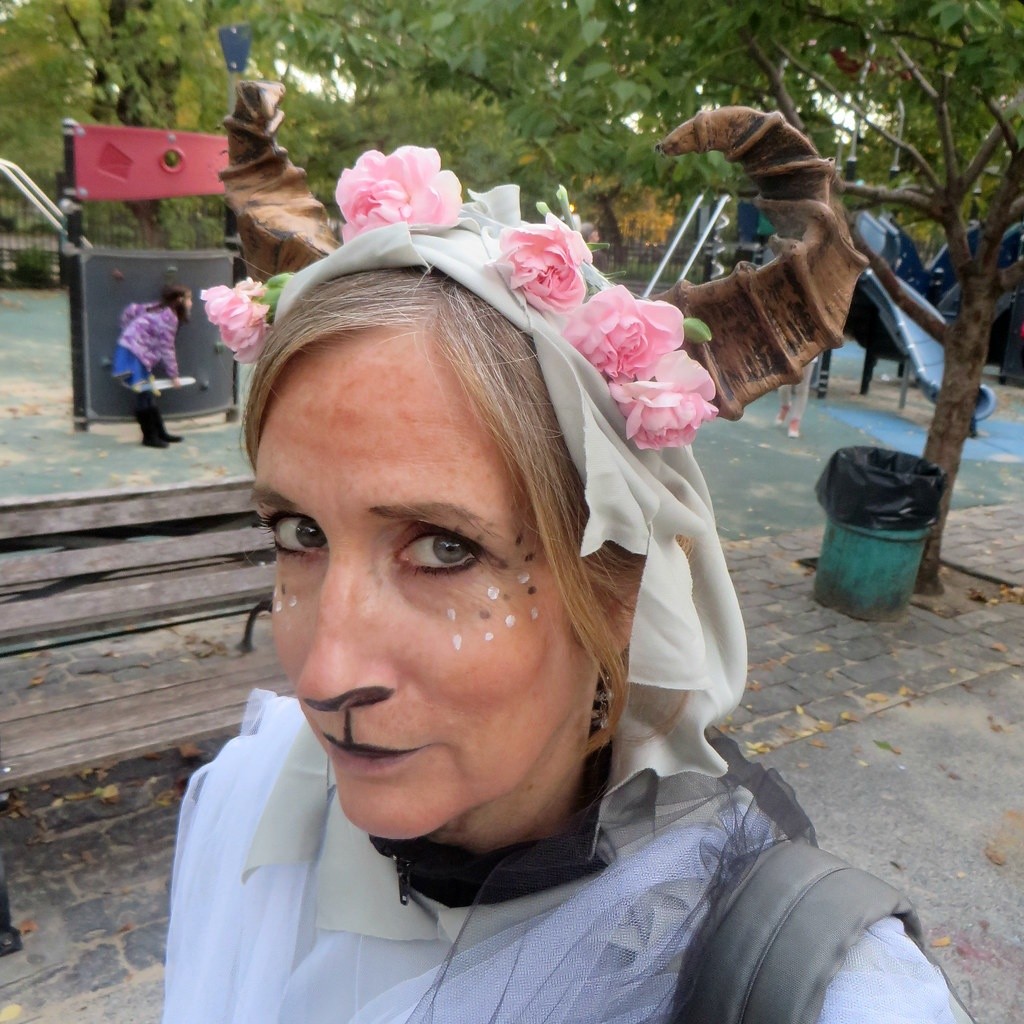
[857,265,997,441]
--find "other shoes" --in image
[775,413,785,426]
[787,427,800,438]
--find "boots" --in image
[153,408,184,442]
[133,406,169,447]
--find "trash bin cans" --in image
[805,443,946,625]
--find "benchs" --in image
[0,479,295,957]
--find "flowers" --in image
[567,286,685,378]
[332,143,458,246]
[606,353,718,453]
[202,269,295,366]
[497,219,593,320]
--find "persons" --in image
[161,81,954,1024]
[769,355,818,438]
[110,283,193,449]
